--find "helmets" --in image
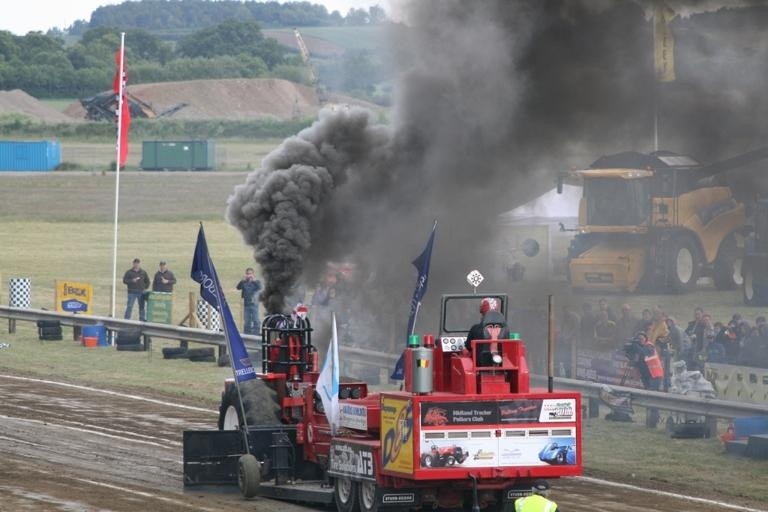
[479,297,500,315]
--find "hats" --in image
[159,260,167,265]
[635,330,649,341]
[132,259,141,265]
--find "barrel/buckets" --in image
[81,324,107,346]
[83,336,99,347]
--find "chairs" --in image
[480,309,509,374]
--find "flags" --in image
[389,226,437,383]
[651,3,678,83]
[315,315,343,436]
[119,94,135,168]
[112,45,128,94]
[191,224,256,384]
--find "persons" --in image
[122,258,151,321]
[558,269,767,428]
[511,480,560,512]
[236,268,262,335]
[310,268,354,310]
[153,261,176,292]
[142,280,153,321]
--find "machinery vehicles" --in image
[551,152,748,297]
[744,190,767,301]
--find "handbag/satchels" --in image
[670,419,709,439]
